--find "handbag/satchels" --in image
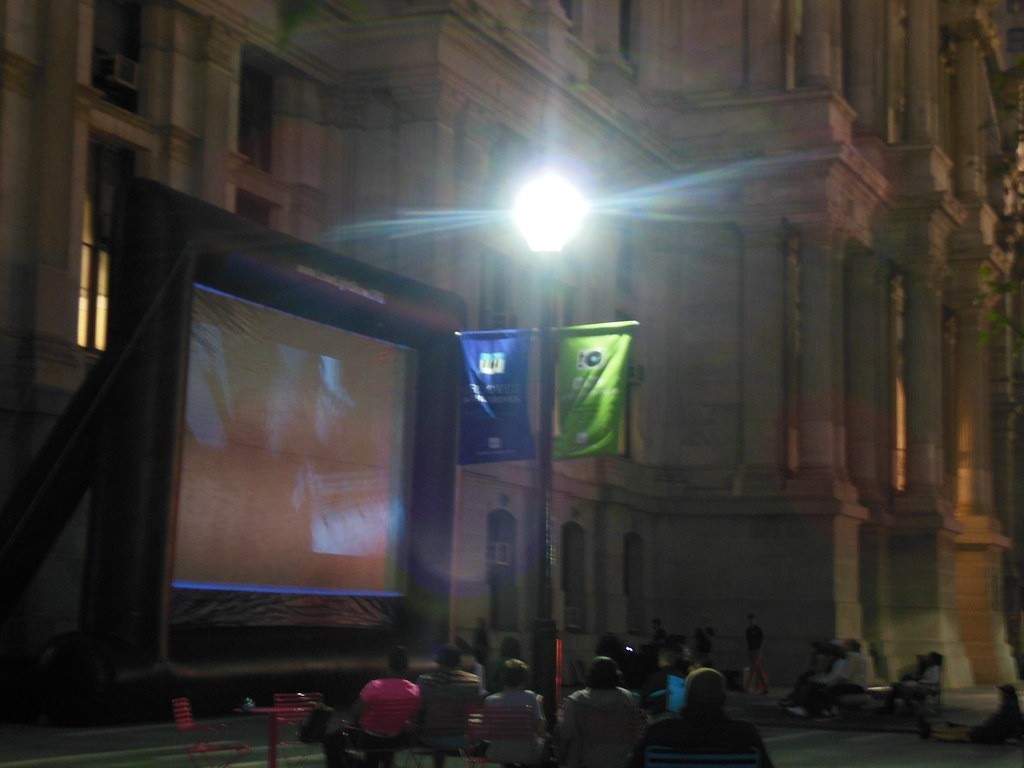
[298,703,334,744]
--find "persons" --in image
[887,652,942,721]
[632,669,776,768]
[319,617,654,768]
[638,615,864,721]
[966,684,1024,745]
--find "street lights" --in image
[468,109,634,757]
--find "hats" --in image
[591,656,624,678]
[686,668,725,705]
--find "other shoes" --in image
[785,705,808,719]
[916,714,932,738]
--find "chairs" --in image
[172,698,252,768]
[269,691,762,768]
